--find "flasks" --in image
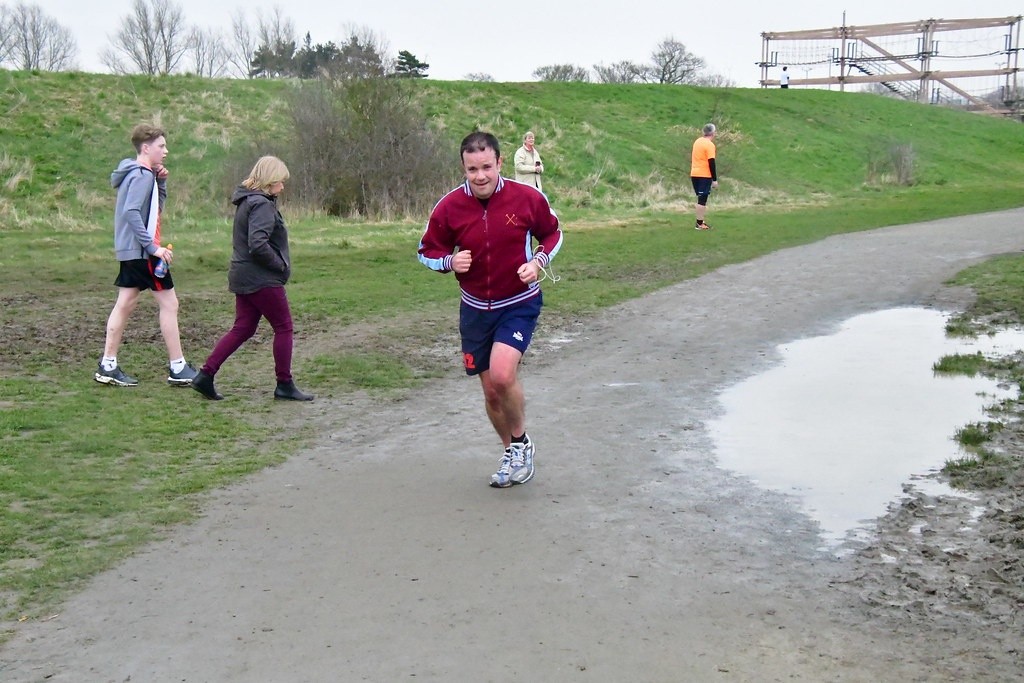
[535,161,540,175]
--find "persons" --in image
[90,121,204,389]
[690,123,719,232]
[780,66,790,89]
[189,154,318,402]
[513,131,545,194]
[416,130,564,490]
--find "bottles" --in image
[154,244,174,278]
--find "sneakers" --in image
[92,362,200,387]
[694,221,712,230]
[488,434,535,488]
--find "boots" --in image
[190,367,314,402]
